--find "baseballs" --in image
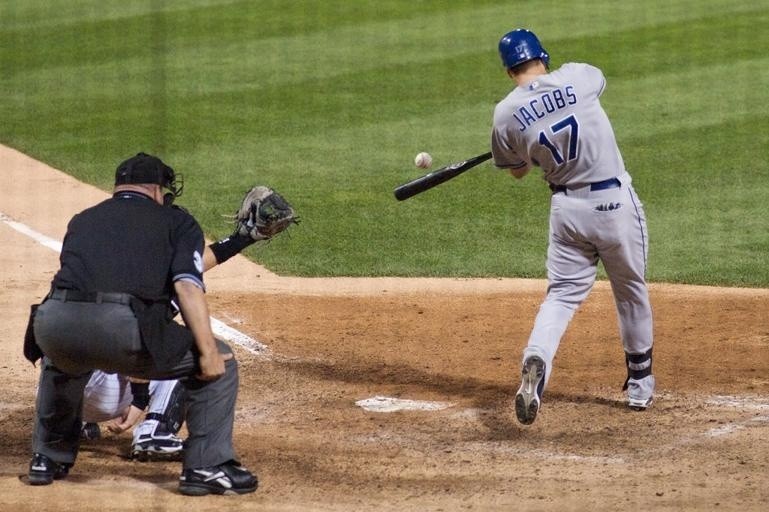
[415,151,432,169]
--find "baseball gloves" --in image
[231,184,302,242]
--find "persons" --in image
[26,153,260,494]
[78,296,191,461]
[491,29,656,424]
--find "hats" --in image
[499,27,549,71]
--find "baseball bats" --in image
[393,150,493,201]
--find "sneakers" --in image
[132,433,184,463]
[515,355,545,425]
[28,453,68,484]
[80,424,99,442]
[629,396,654,408]
[178,464,258,497]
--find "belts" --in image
[549,177,622,192]
[49,289,132,308]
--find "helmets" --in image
[112,152,185,206]
